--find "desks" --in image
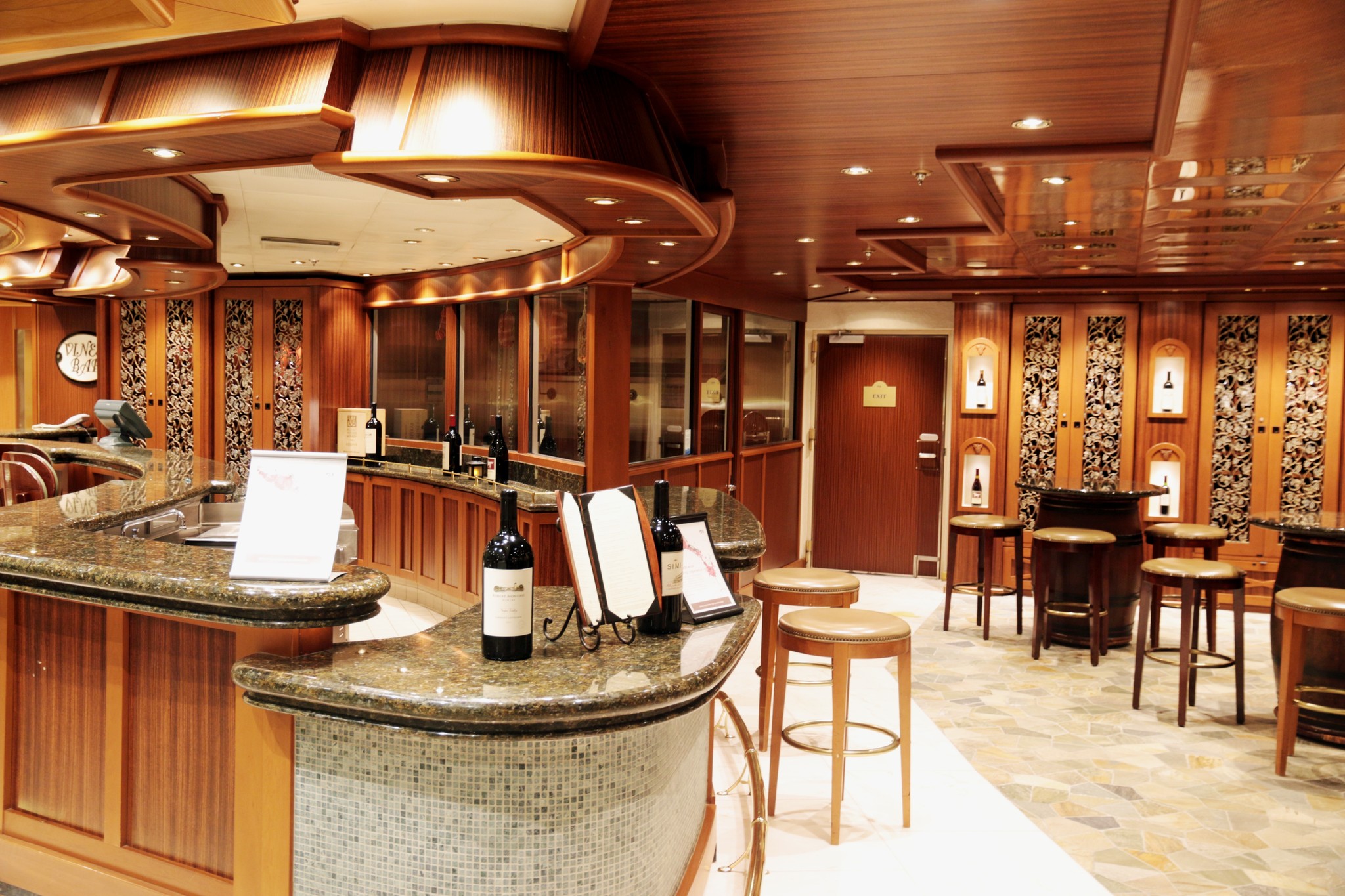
[1010,476,1167,650]
[1246,509,1345,747]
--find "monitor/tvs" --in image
[93,399,154,441]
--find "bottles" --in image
[481,487,534,662]
[1160,475,1169,514]
[976,369,987,409]
[423,405,440,443]
[1163,370,1174,411]
[972,468,982,505]
[488,414,509,485]
[539,416,558,457]
[442,414,463,476]
[365,402,382,467]
[462,402,476,447]
[634,480,684,636]
[537,404,545,450]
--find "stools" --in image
[1030,527,1117,667]
[765,607,916,848]
[1132,556,1249,728]
[942,514,1026,640]
[1271,585,1345,777]
[1143,520,1228,655]
[748,566,862,753]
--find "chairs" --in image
[0,451,60,507]
